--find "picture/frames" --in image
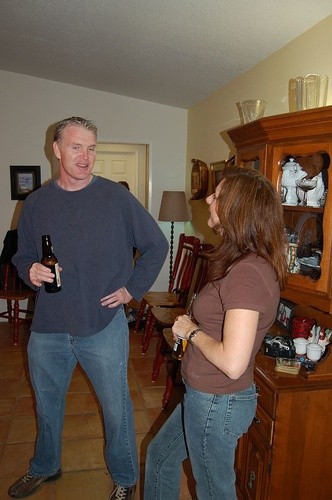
[10,165,40,199]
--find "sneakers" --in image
[109,483,136,500]
[8,468,62,498]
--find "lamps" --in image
[158,190,192,289]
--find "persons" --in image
[144,168,289,500]
[8,116,169,500]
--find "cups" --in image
[41,235,61,293]
[285,232,300,272]
[172,293,196,360]
[293,338,323,362]
[240,99,266,125]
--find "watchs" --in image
[189,329,203,343]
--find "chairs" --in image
[134,233,215,409]
[0,231,35,345]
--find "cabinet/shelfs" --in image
[235,324,332,500]
[228,105,332,315]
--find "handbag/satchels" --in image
[264,336,295,359]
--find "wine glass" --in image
[296,179,317,207]
